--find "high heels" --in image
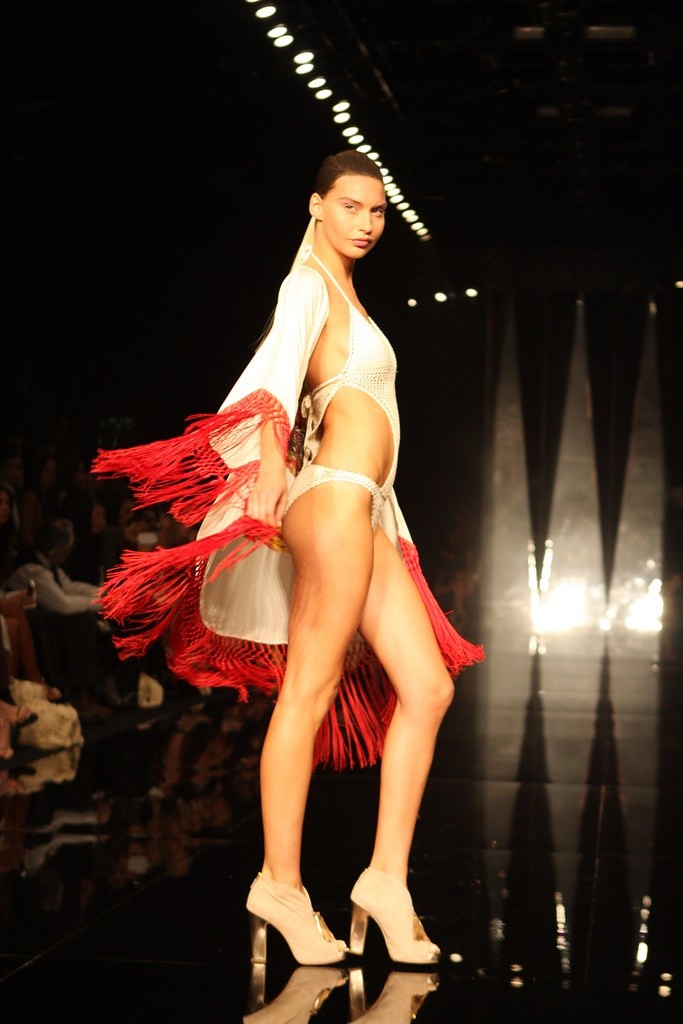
[349,966,440,1024]
[349,867,440,963]
[246,872,344,965]
[243,964,349,1024]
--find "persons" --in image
[0,446,185,778]
[241,150,486,968]
